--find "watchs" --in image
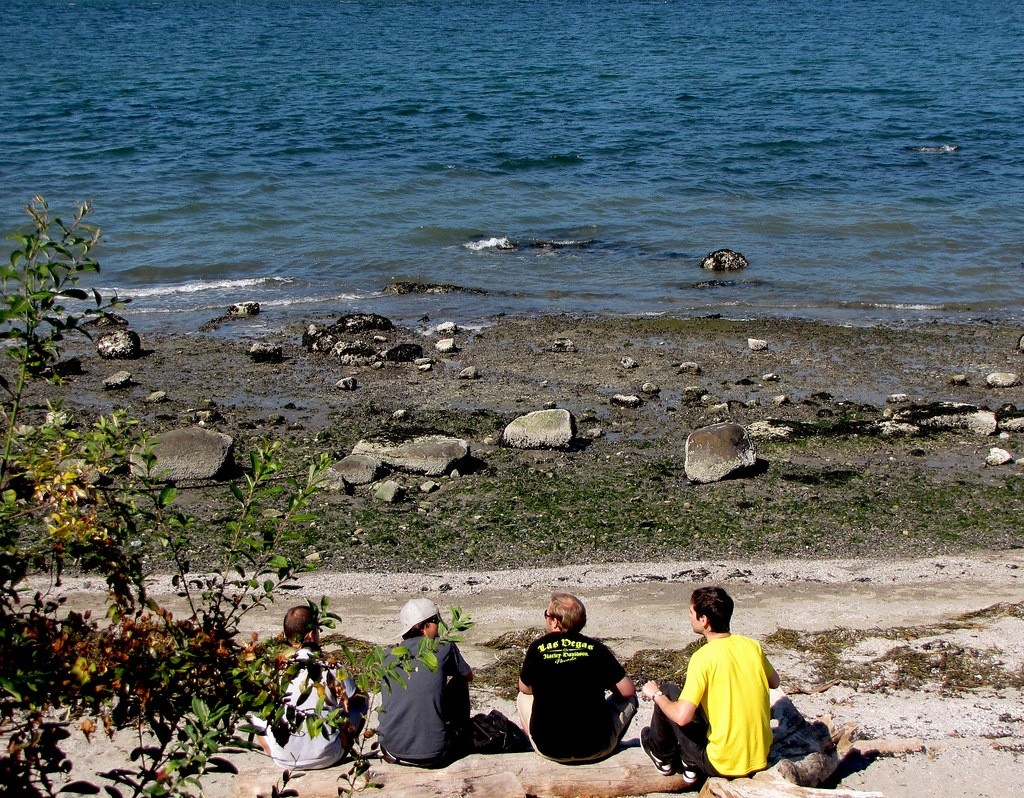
[652,691,663,700]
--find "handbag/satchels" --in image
[468,710,529,753]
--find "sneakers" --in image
[682,759,697,783]
[640,725,672,776]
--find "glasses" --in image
[544,609,556,620]
[418,618,439,628]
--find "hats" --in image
[395,597,440,639]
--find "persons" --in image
[640,585,783,783]
[256,605,369,770]
[380,598,474,768]
[518,594,638,764]
